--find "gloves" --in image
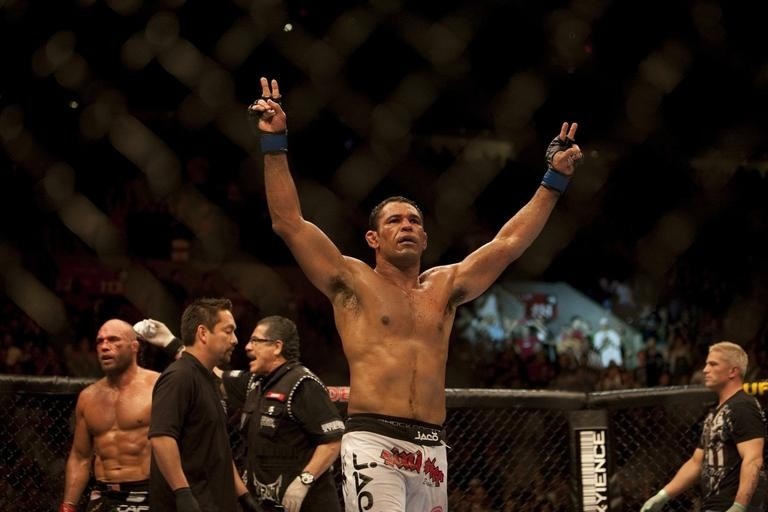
[282,476,310,512]
[725,502,746,512]
[143,318,176,347]
[173,487,199,511]
[238,491,262,512]
[641,488,668,512]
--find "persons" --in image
[0,260,767,512]
[244,73,587,512]
